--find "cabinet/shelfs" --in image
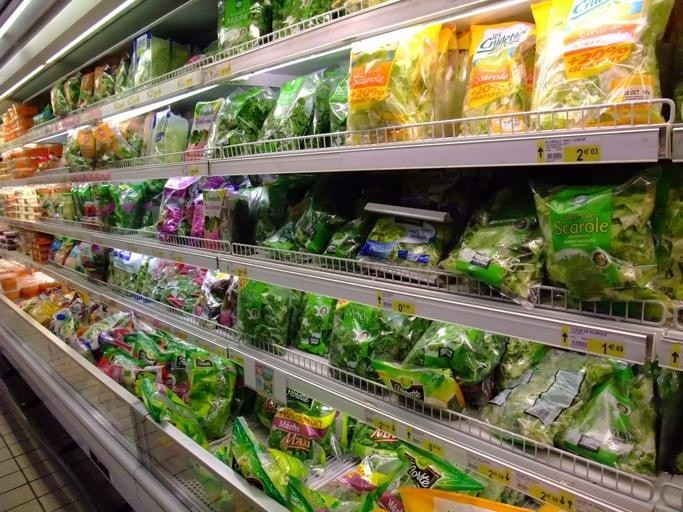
[0,1,683,512]
